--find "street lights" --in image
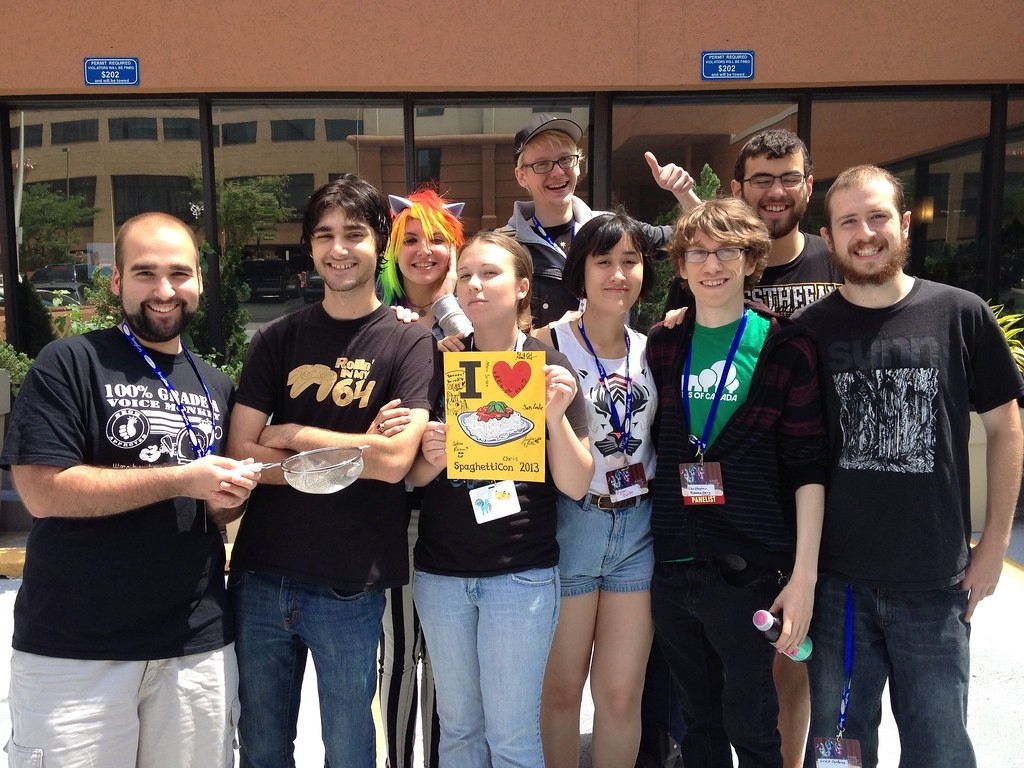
[65,145,70,200]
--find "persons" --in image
[1,212,262,767]
[224,174,439,768]
[375,113,1024,768]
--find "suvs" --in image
[25,262,92,307]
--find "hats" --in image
[514,114,583,167]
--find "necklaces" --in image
[407,298,432,317]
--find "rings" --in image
[376,423,386,433]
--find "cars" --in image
[235,255,325,305]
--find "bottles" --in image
[752,610,815,663]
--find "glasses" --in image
[683,248,749,264]
[523,154,580,174]
[740,174,806,189]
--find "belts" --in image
[581,493,651,510]
